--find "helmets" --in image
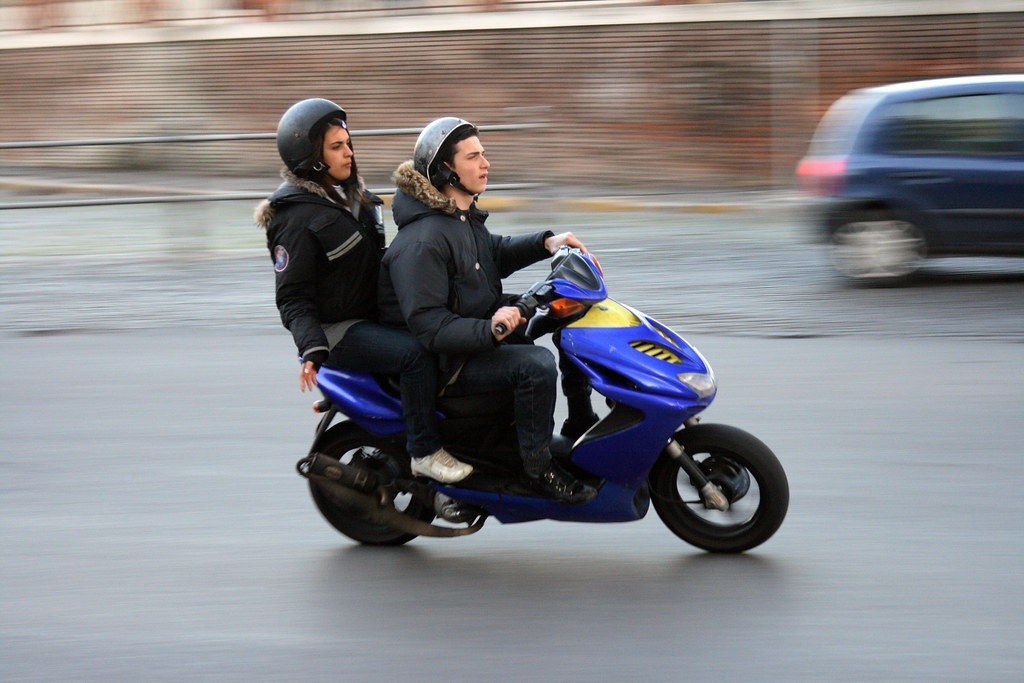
[413,117,475,184]
[277,98,348,174]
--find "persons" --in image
[376,115,598,504]
[253,97,474,484]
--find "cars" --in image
[792,74,1023,286]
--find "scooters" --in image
[292,240,791,560]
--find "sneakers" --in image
[410,448,473,484]
[518,468,596,504]
[559,412,598,443]
